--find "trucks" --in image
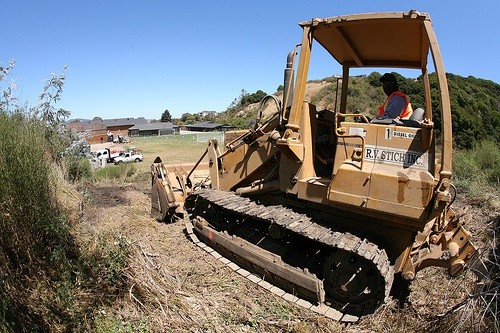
[96,147,128,161]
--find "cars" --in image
[113,150,144,163]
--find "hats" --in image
[380,73,396,82]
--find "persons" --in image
[377,72,413,119]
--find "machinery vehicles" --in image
[151,8,489,321]
